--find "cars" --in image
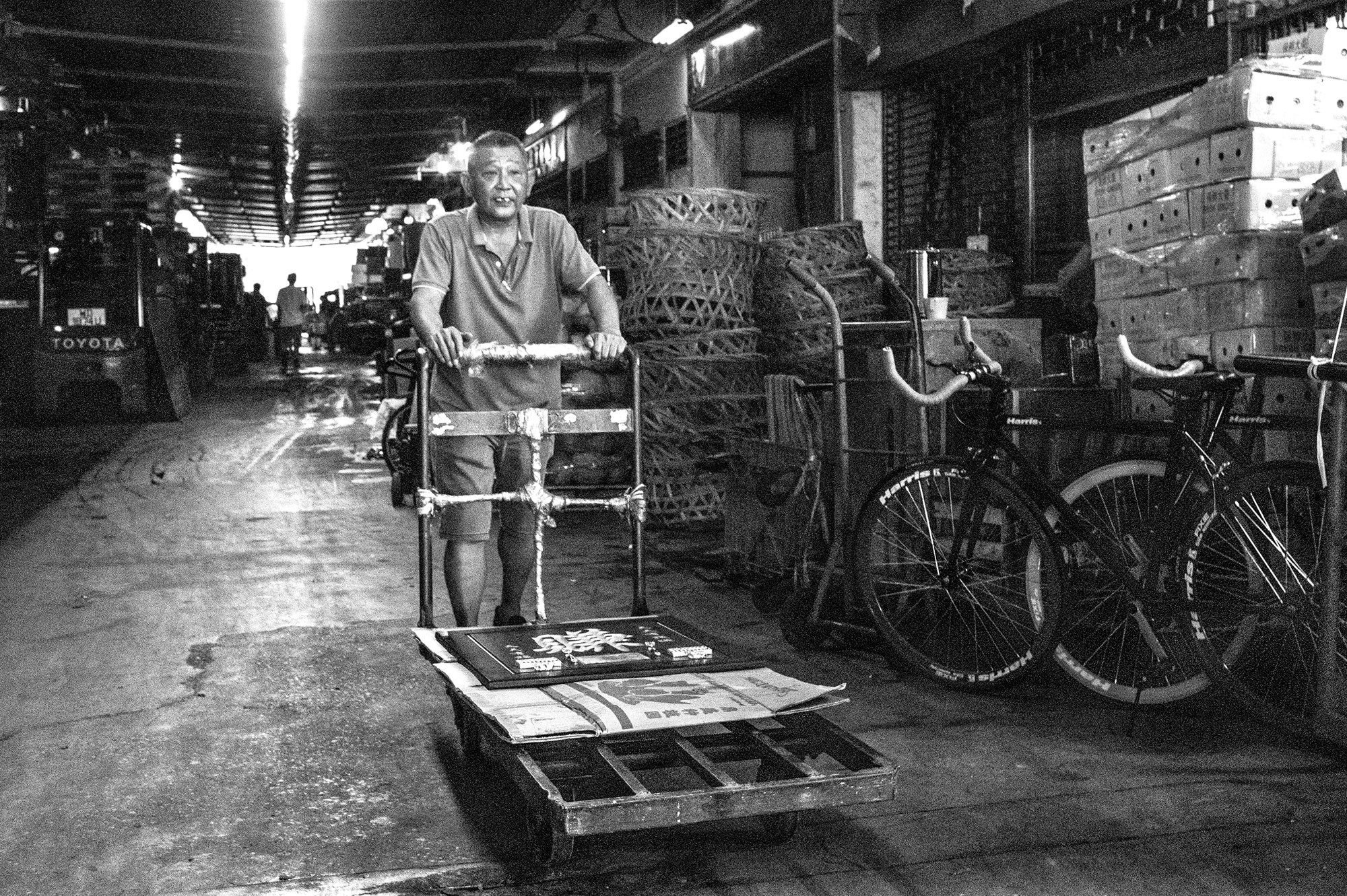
[324,294,405,353]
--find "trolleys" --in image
[410,341,902,863]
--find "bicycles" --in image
[1017,333,1346,715]
[852,316,1347,749]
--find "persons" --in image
[277,273,306,376]
[252,283,276,310]
[409,130,628,625]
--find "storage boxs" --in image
[1083,18,1347,463]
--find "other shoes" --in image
[493,605,527,626]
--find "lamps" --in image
[652,15,695,45]
[525,116,548,135]
